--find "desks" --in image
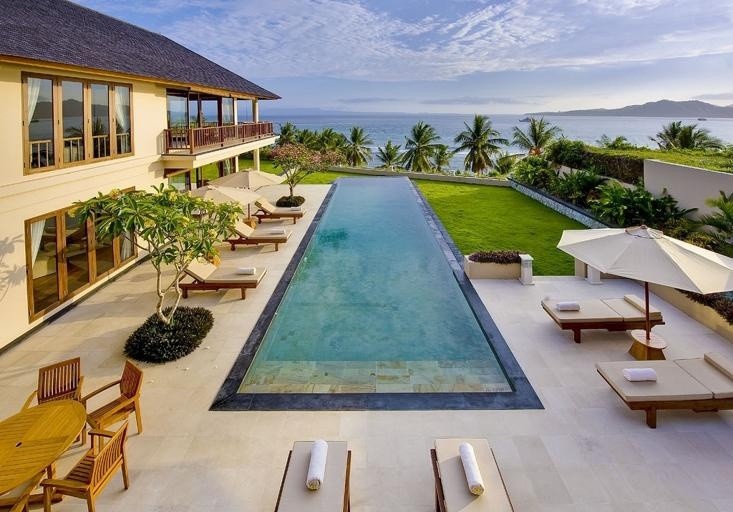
[628,328,668,360]
[0,398,87,509]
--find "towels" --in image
[557,302,580,311]
[306,440,328,490]
[459,442,484,496]
[271,229,285,234]
[239,268,255,275]
[623,368,657,382]
[291,207,301,211]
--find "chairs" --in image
[541,294,665,343]
[8,420,130,512]
[596,351,733,428]
[251,197,307,224]
[21,356,85,442]
[430,438,515,512]
[179,254,267,299]
[79,359,144,448]
[274,440,351,512]
[222,220,293,251]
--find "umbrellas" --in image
[556,225,733,341]
[191,167,287,221]
